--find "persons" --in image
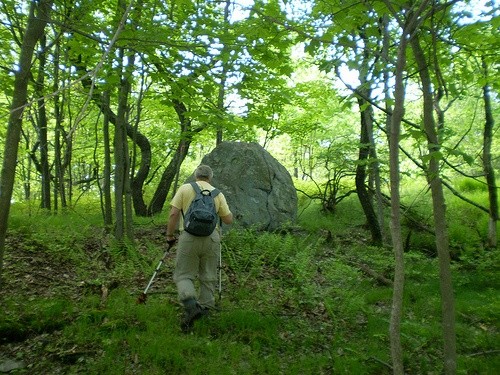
[165,165,233,334]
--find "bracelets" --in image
[165,234,174,237]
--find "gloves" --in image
[167,237,176,246]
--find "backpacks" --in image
[180,181,221,236]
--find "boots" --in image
[179,296,204,332]
